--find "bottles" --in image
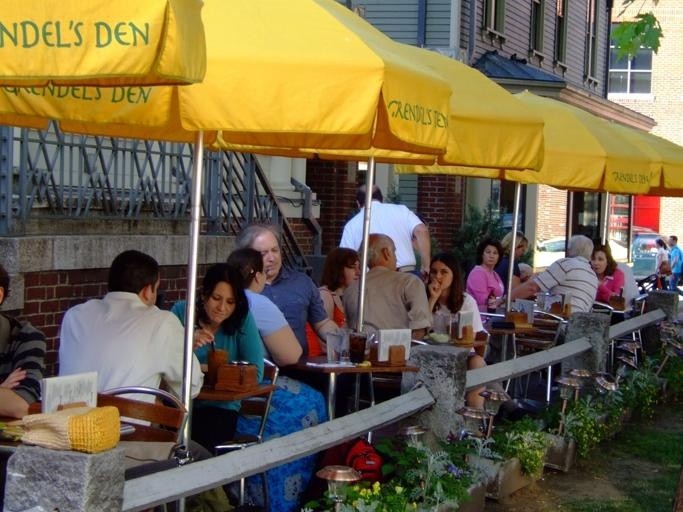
[486,288,497,314]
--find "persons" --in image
[58,249,231,512]
[0,268,45,417]
[169,262,267,502]
[339,183,432,287]
[488,235,598,314]
[465,231,528,325]
[668,236,683,296]
[652,239,670,291]
[225,248,327,511]
[590,246,624,304]
[316,233,486,411]
[234,224,340,383]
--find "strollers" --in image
[636,272,663,294]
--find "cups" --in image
[448,314,458,339]
[206,349,228,386]
[326,330,379,366]
[536,293,549,310]
[327,480,347,502]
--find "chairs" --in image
[0,369,183,495]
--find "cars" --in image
[534,237,630,268]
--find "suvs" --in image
[624,232,663,279]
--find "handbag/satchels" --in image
[660,262,671,273]
[319,434,380,482]
[19,407,123,452]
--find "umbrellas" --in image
[212,40,545,411]
[1,0,207,87]
[617,122,683,264]
[394,86,651,361]
[0,0,452,512]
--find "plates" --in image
[315,465,364,482]
[454,319,683,435]
[400,425,426,435]
